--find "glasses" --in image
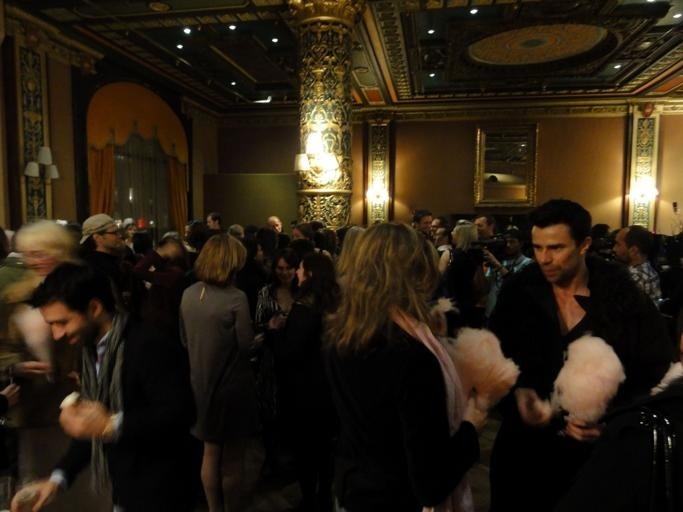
[104,229,119,235]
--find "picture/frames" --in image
[70,48,194,226]
[473,119,542,208]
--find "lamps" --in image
[25,146,60,189]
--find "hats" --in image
[78,214,116,245]
